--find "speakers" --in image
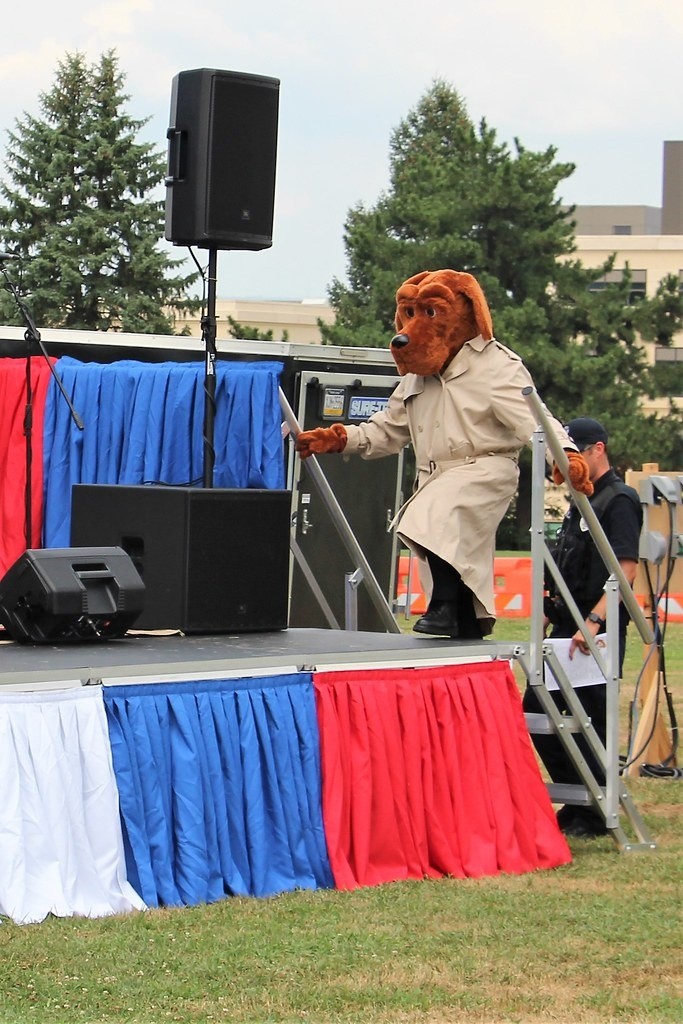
[68,484,294,634]
[0,545,147,646]
[164,67,280,251]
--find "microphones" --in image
[0,252,21,261]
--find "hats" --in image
[563,417,608,453]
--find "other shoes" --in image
[556,805,579,830]
[565,814,607,839]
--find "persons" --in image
[523,420,643,840]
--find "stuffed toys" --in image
[298,270,594,642]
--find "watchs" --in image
[589,611,604,626]
